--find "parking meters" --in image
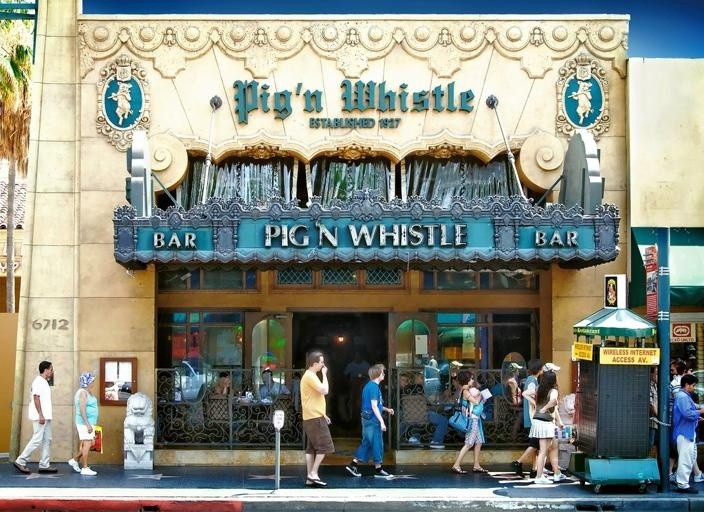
[270,408,284,490]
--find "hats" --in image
[509,363,523,373]
[79,372,96,389]
[449,361,464,370]
[543,363,561,373]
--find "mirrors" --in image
[100,357,138,406]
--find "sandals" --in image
[471,466,489,473]
[451,465,467,474]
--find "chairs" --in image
[178,395,294,443]
[400,394,526,445]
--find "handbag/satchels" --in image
[448,411,473,433]
[362,409,372,421]
[80,426,103,454]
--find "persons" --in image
[299,353,336,486]
[253,370,291,400]
[346,364,395,479]
[397,361,574,485]
[67,372,98,476]
[428,356,438,368]
[210,372,230,399]
[12,361,59,475]
[649,358,704,494]
[342,351,369,380]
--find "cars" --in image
[164,356,286,408]
[418,363,442,398]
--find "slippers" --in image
[305,476,328,488]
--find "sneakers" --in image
[68,458,98,476]
[345,464,362,477]
[511,461,566,484]
[677,471,704,494]
[13,461,31,474]
[39,466,58,473]
[374,470,394,478]
